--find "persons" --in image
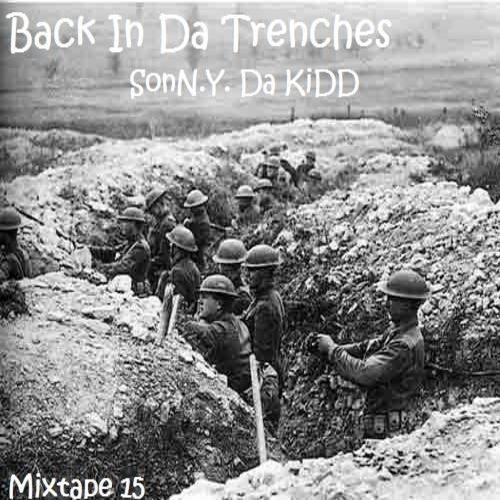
[0,206,31,283]
[63,139,322,428]
[304,270,430,440]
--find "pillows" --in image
[0,205,27,233]
[376,268,435,300]
[241,243,284,268]
[232,185,257,199]
[252,178,276,192]
[141,186,170,213]
[163,223,202,256]
[192,274,240,300]
[181,188,209,208]
[115,206,146,223]
[210,238,249,264]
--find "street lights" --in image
[361,411,407,436]
[242,357,282,433]
